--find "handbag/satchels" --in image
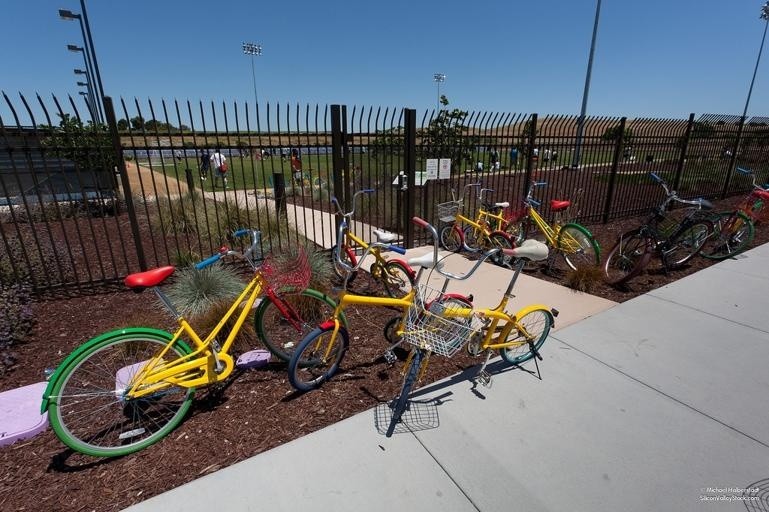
[495,163,500,170]
[543,157,549,162]
[219,164,228,172]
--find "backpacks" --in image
[492,150,497,162]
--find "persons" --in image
[198,146,228,189]
[290,149,304,184]
[474,141,558,172]
[238,145,288,162]
[176,149,181,165]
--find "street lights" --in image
[58,0,110,128]
[242,39,263,136]
[722,2,769,195]
[431,71,448,131]
